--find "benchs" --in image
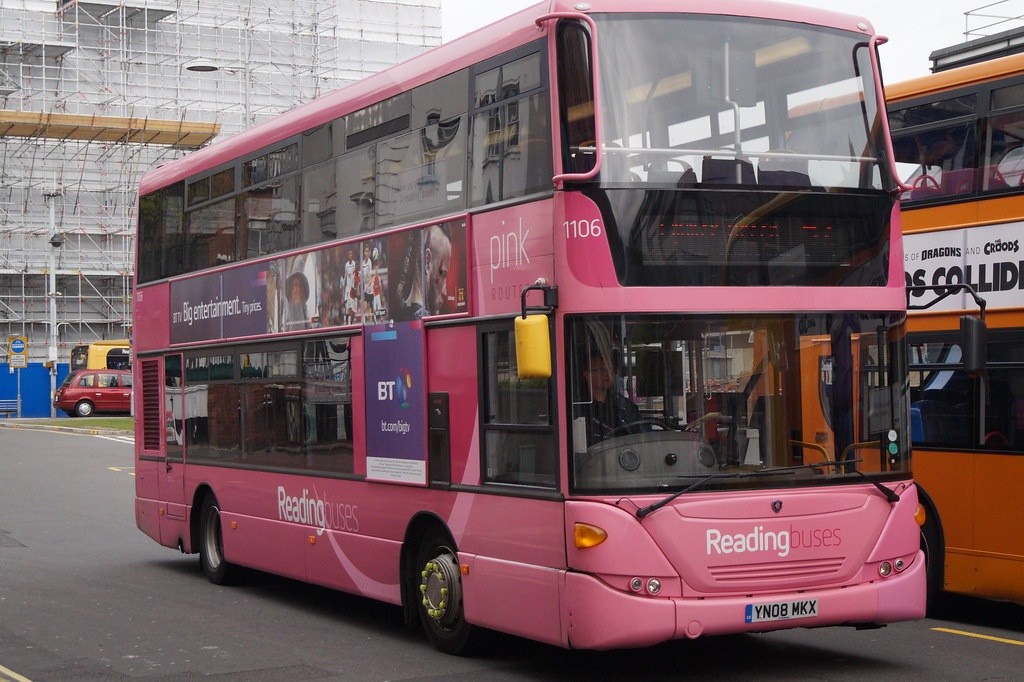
[0,400,18,418]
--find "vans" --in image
[53,368,134,416]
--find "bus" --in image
[130,2,995,652]
[69,338,133,374]
[679,21,1024,619]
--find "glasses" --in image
[589,366,612,373]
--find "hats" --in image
[285,255,310,302]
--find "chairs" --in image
[163,135,1024,473]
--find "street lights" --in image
[180,57,252,131]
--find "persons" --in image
[572,350,653,446]
[400,225,453,319]
[342,245,383,328]
[282,272,310,333]
